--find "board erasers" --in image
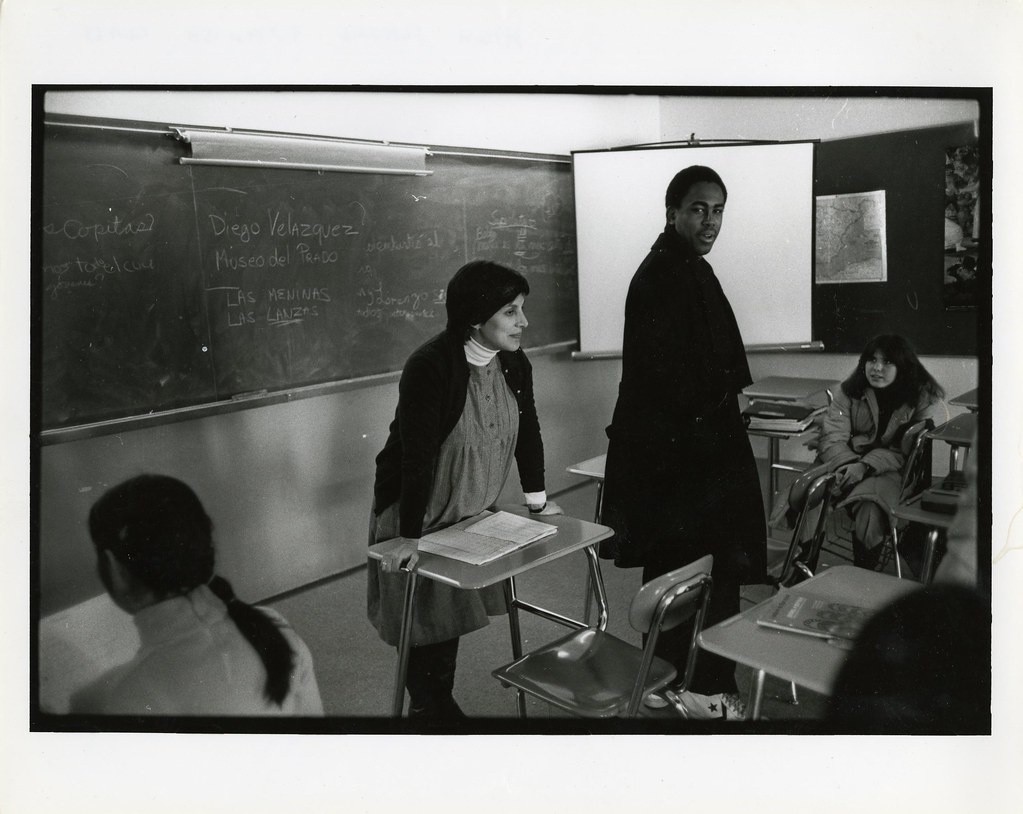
[233,389,268,400]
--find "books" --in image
[417,510,559,565]
[756,585,879,641]
[921,469,966,514]
[742,402,818,432]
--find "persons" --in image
[594,166,771,721]
[66,473,326,716]
[776,335,948,596]
[365,259,566,714]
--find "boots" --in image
[782,539,821,588]
[852,530,886,571]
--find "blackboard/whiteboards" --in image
[812,117,978,361]
[41,111,580,445]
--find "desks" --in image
[361,503,615,719]
[890,386,979,586]
[565,450,606,549]
[740,374,842,494]
[694,561,929,720]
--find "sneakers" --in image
[674,687,769,722]
[645,692,670,709]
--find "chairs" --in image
[763,421,933,591]
[490,553,715,718]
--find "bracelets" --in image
[528,503,546,513]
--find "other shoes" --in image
[409,693,483,734]
